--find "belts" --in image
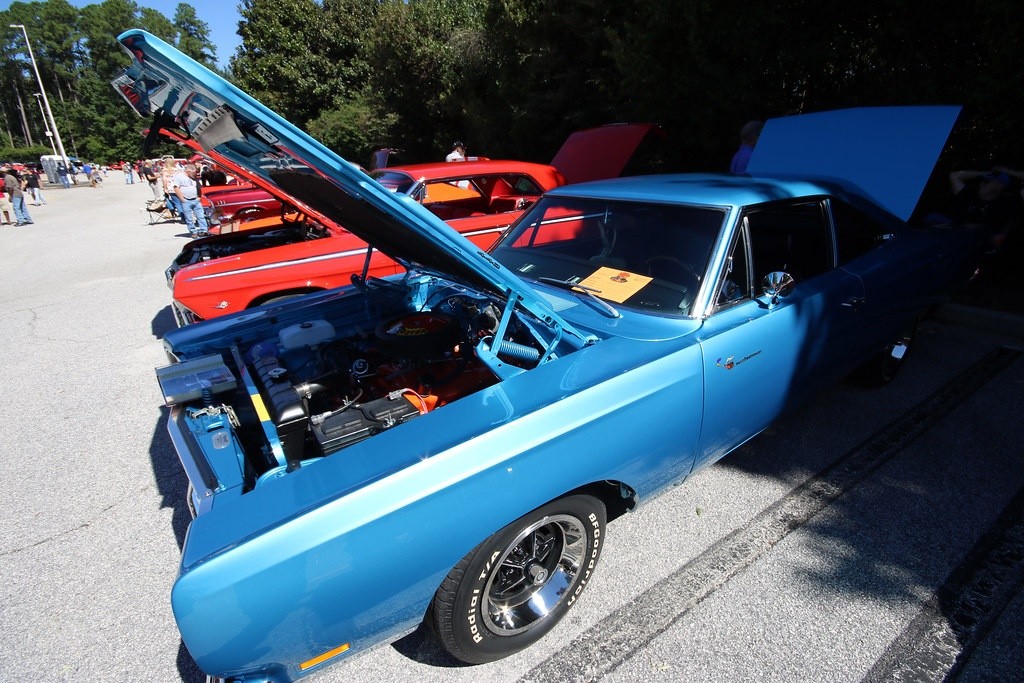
[185,198,196,200]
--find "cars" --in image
[110,27,964,683]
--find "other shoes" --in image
[198,232,208,237]
[180,220,186,224]
[24,221,34,225]
[191,234,198,239]
[14,223,24,227]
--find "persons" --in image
[120,157,228,238]
[56,161,79,189]
[83,162,110,185]
[0,165,47,227]
[446,140,474,189]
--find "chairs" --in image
[146,194,169,224]
[669,228,714,289]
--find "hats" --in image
[452,142,467,150]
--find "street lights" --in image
[9,22,79,185]
